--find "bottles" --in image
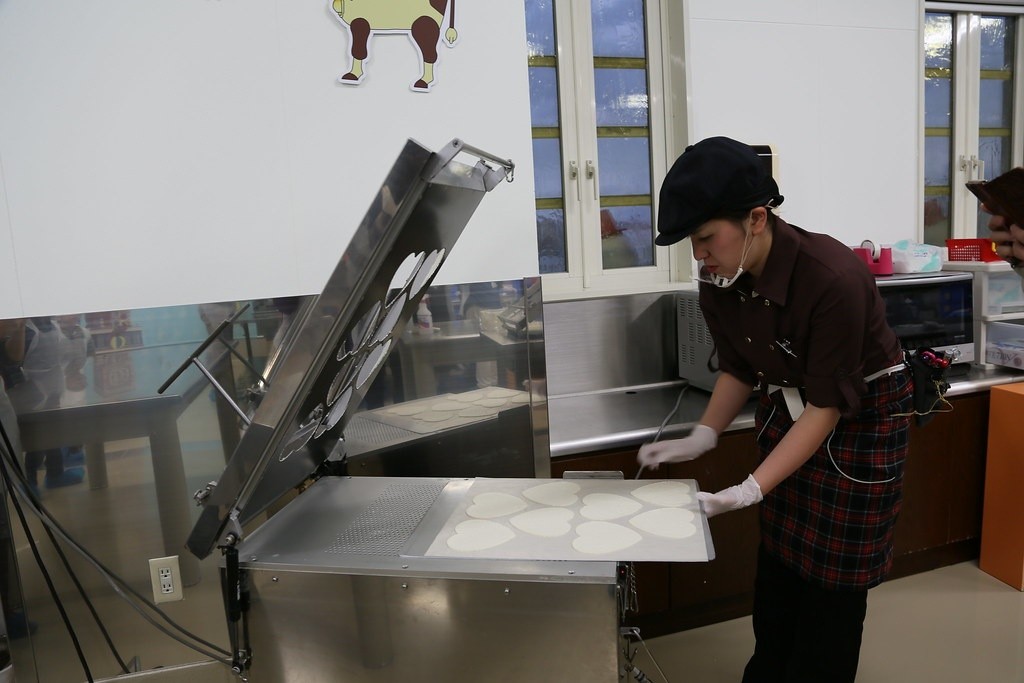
[499,282,518,308]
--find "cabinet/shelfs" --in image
[548,389,989,646]
[977,379,1024,592]
[942,256,1024,370]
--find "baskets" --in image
[945,238,1005,262]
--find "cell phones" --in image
[966,166,1024,231]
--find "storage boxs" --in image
[945,237,1004,262]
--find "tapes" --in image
[860,237,882,260]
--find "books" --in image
[964,166,1024,231]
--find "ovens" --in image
[858,270,974,368]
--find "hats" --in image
[655,136,773,246]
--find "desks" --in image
[6,335,243,591]
[398,317,542,401]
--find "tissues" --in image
[891,240,944,272]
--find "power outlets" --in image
[148,553,186,607]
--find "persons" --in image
[637,137,912,683]
[978,203,1024,296]
